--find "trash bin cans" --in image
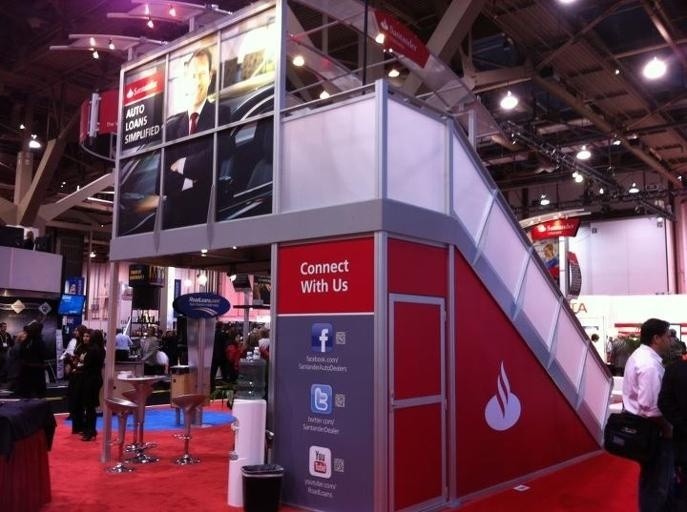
[240,463,284,512]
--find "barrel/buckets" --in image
[237,358,266,400]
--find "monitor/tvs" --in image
[56,293,86,315]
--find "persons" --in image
[0,320,269,441]
[589,319,687,511]
[24,231,34,250]
[69,281,77,294]
[155,47,236,230]
[543,244,559,286]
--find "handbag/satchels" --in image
[604,412,661,462]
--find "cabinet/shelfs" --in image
[129,285,161,338]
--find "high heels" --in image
[82,431,98,441]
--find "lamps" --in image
[147,14,154,30]
[500,80,519,111]
[539,193,551,206]
[168,4,176,17]
[28,133,42,150]
[576,145,591,161]
[388,62,400,78]
[656,214,664,228]
[107,40,115,51]
[92,51,100,60]
[627,182,641,194]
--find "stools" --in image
[122,387,156,451]
[172,394,210,467]
[105,396,136,473]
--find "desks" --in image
[0,397,58,510]
[117,372,166,465]
